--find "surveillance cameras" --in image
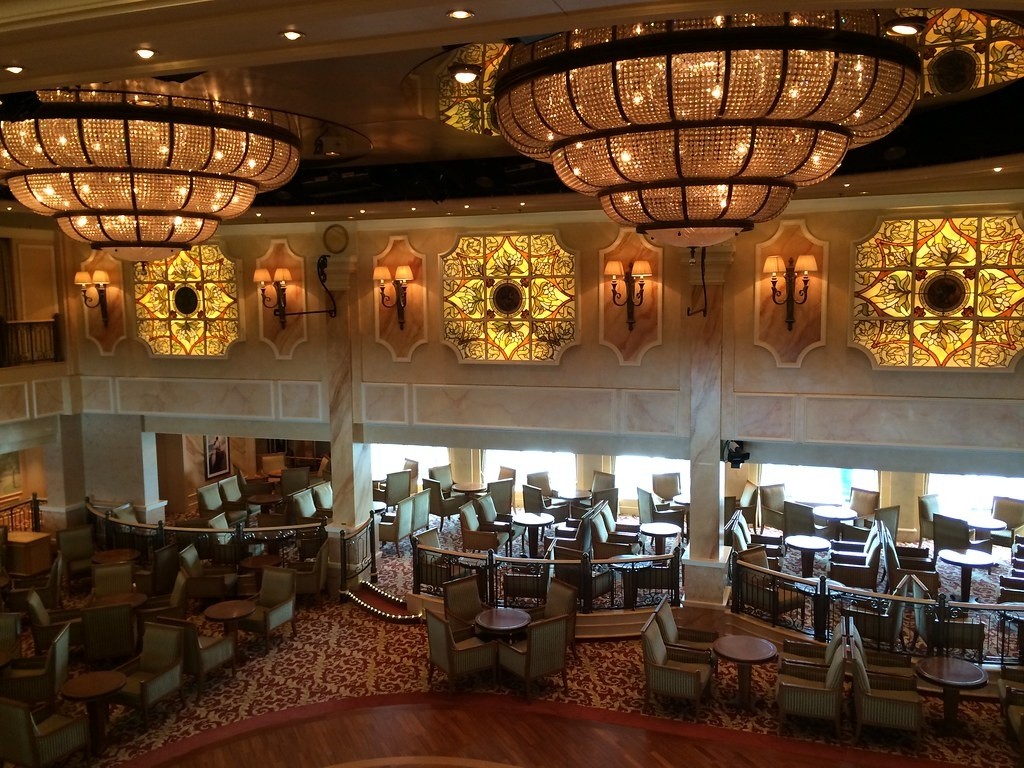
[723,439,741,454]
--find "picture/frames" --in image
[203,435,231,480]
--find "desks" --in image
[91,548,142,564]
[475,607,531,644]
[239,554,282,592]
[63,670,126,752]
[640,521,682,566]
[452,481,486,502]
[812,505,858,520]
[713,634,778,704]
[248,493,283,513]
[267,467,288,479]
[245,529,295,556]
[795,577,846,596]
[80,592,148,653]
[785,534,831,577]
[916,655,989,734]
[608,555,652,605]
[204,599,257,659]
[939,548,995,604]
[966,515,1008,540]
[370,470,387,482]
[673,493,690,541]
[555,488,592,517]
[133,523,159,558]
[456,550,502,602]
[512,512,555,559]
[998,601,1024,655]
[373,499,386,513]
[0,574,12,611]
[4,527,52,577]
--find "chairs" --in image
[0,451,1024,768]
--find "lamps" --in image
[884,15,931,36]
[493,13,921,248]
[373,265,414,330]
[75,269,111,327]
[447,63,483,84]
[762,254,818,330]
[255,267,292,328]
[604,261,652,330]
[0,77,301,265]
